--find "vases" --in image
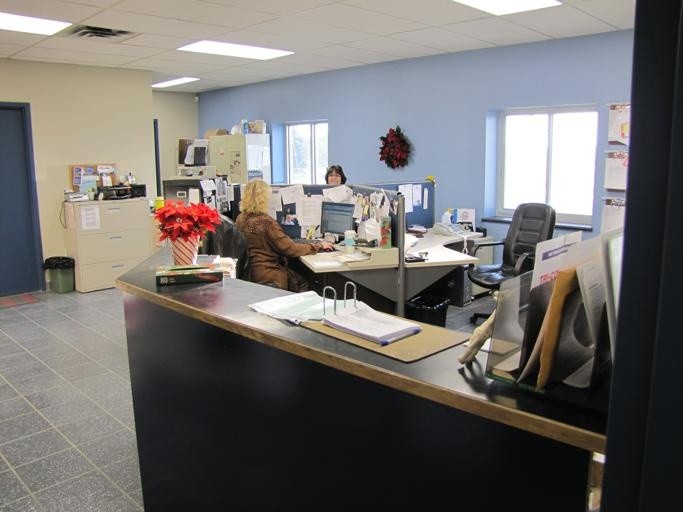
[172,235,199,267]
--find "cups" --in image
[344,230,356,246]
[241,120,249,134]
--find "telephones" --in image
[433,222,463,236]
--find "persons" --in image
[232,178,336,293]
[323,165,348,186]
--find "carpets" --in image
[0,292,41,309]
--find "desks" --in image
[299,231,483,318]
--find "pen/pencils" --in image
[304,224,319,240]
[88,186,95,192]
[381,328,420,346]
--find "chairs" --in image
[467,202,556,324]
[198,213,279,288]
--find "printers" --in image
[98,185,132,200]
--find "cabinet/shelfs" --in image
[62,197,154,294]
[446,236,496,300]
[208,133,272,184]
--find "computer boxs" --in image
[446,267,472,306]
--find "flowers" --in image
[378,125,411,171]
[155,198,223,242]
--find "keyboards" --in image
[293,237,320,243]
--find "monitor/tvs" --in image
[320,202,355,242]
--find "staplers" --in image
[352,238,369,247]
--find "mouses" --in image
[325,247,335,252]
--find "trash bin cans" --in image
[45,256,75,294]
[404,294,450,328]
